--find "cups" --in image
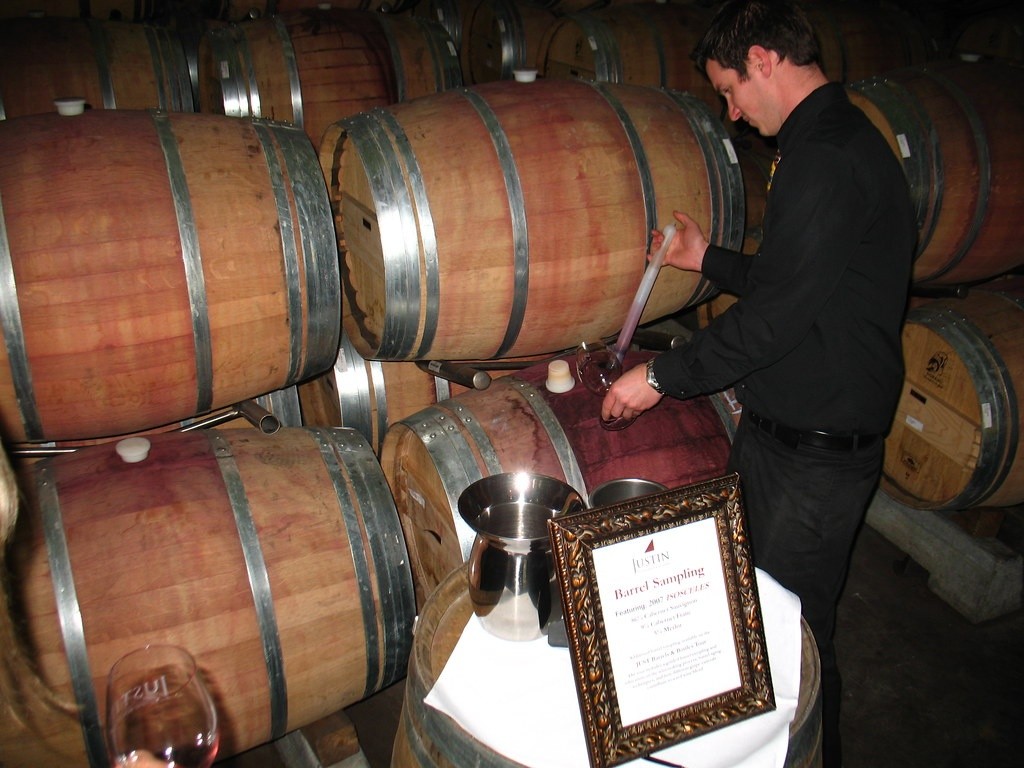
[108,644,218,768]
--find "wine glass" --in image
[577,341,640,429]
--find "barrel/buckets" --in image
[0,1,1024,768]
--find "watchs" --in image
[646,357,666,396]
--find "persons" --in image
[600,0,919,768]
